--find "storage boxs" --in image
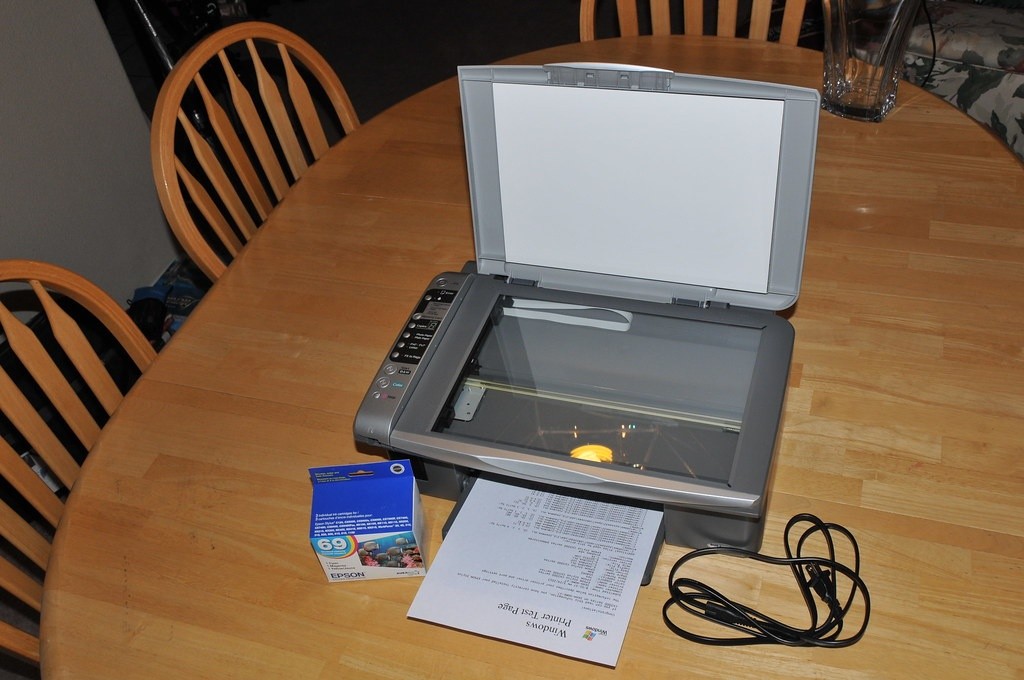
[308,459,426,583]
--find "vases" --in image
[820,0,922,124]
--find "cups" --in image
[819,0,919,123]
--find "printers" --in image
[352,62,820,553]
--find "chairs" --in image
[579,0,807,46]
[0,259,157,663]
[151,22,361,284]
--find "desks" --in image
[40,36,1024,680]
